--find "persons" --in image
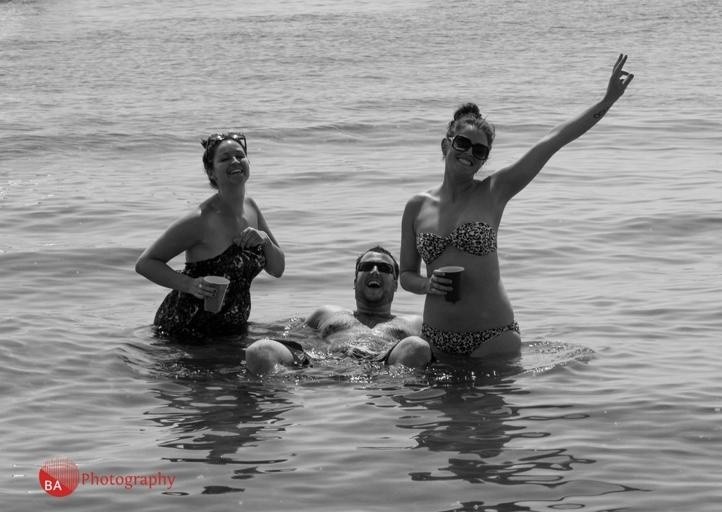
[133,129,286,349]
[245,244,433,375]
[399,51,636,365]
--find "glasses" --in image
[447,134,490,161]
[206,132,248,166]
[356,261,396,280]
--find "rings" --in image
[197,283,203,289]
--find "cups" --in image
[436,264,465,304]
[203,274,231,314]
